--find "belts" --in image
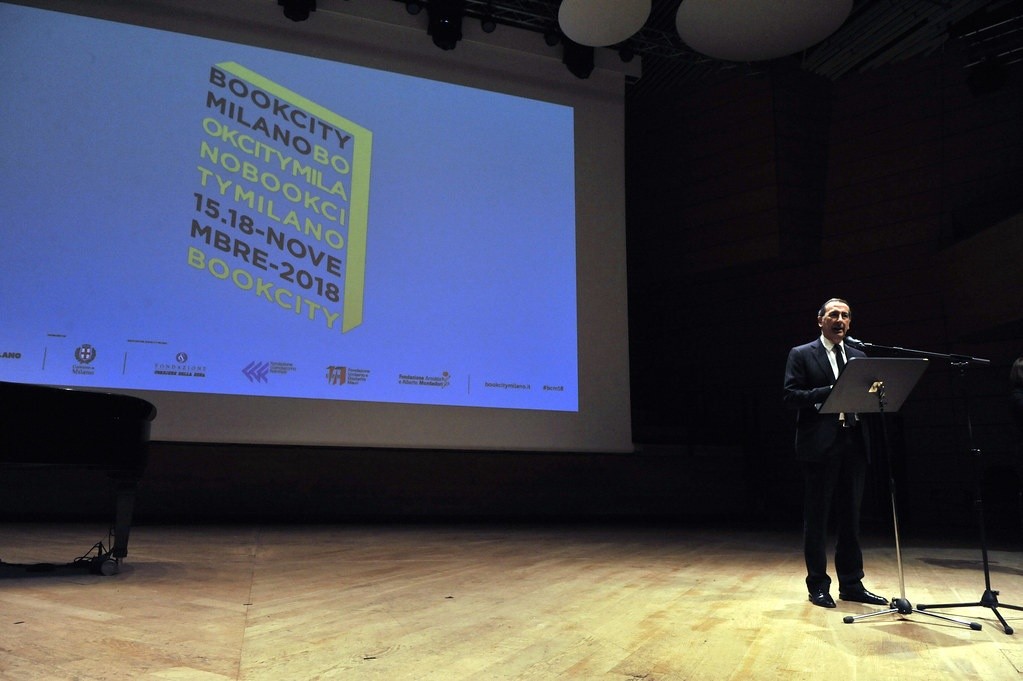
[837,420,861,432]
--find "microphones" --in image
[845,336,869,350]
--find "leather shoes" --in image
[839,582,889,605]
[809,586,836,608]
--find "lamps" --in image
[543,29,559,46]
[482,0,497,31]
[620,47,634,61]
[561,38,595,81]
[425,0,463,50]
[278,0,317,22]
[405,0,422,14]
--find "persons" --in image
[1009,357,1023,430]
[782,296,889,609]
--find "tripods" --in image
[813,344,1023,635]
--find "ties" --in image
[834,344,856,428]
